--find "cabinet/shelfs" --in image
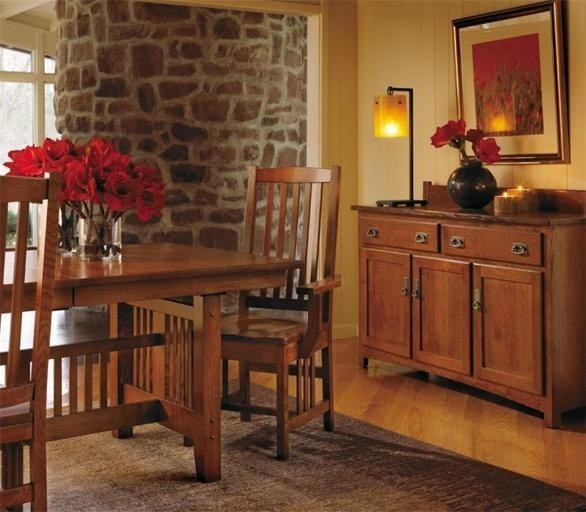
[350,181,586,429]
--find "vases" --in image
[75,216,123,265]
[447,161,499,214]
[57,215,82,258]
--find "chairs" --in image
[184,165,342,460]
[0,172,60,512]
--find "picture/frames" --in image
[450,0,571,164]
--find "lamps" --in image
[373,87,429,209]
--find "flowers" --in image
[59,137,167,257]
[430,120,502,165]
[3,138,88,251]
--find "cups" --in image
[55,213,87,257]
[78,218,122,262]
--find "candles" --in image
[492,194,519,218]
[506,187,543,215]
